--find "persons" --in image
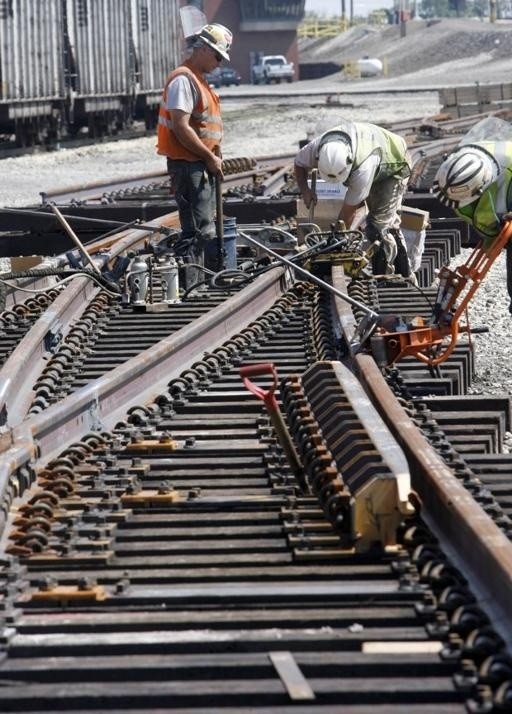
[294,122,418,287]
[158,22,233,283]
[433,139,512,314]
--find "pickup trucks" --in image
[251,54,295,84]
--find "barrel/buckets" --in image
[204,218,241,286]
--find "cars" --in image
[203,67,241,88]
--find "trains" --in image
[0,0,183,153]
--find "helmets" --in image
[439,148,493,203]
[318,141,353,183]
[195,23,233,62]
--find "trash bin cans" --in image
[204,217,238,277]
[344,58,362,82]
